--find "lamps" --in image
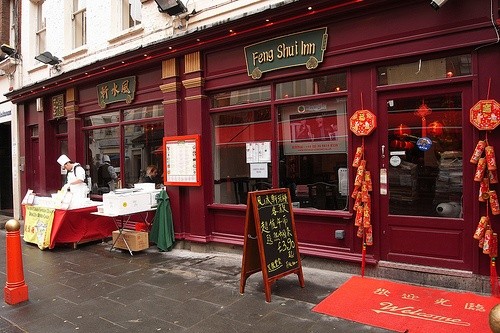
[155,0,188,16]
[1,44,18,58]
[35,52,61,65]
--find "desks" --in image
[22,200,117,249]
[90,208,158,256]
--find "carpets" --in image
[311,276,500,333]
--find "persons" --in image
[311,115,334,141]
[297,120,312,142]
[97,155,118,192]
[57,153,86,191]
[139,165,164,188]
[415,141,440,217]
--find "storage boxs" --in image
[97,182,162,215]
[113,229,149,251]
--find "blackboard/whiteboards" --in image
[241,187,301,282]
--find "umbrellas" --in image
[149,188,175,251]
